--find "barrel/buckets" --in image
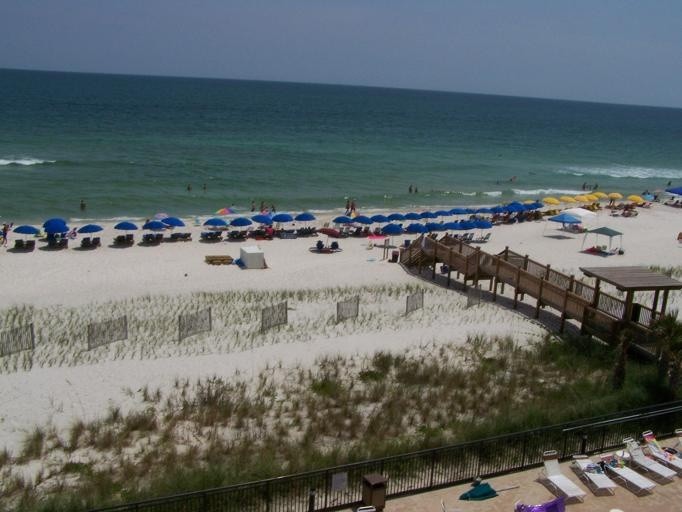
[405,240,410,246]
[392,251,399,260]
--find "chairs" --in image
[534,428,682,502]
[46,238,67,251]
[143,233,191,246]
[16,239,35,251]
[114,234,133,247]
[228,228,315,242]
[81,237,101,249]
[201,231,222,242]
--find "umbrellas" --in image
[43,213,316,241]
[12,225,41,234]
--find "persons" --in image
[187,184,192,193]
[79,199,87,211]
[264,224,273,239]
[642,190,650,195]
[2,223,9,244]
[592,183,599,191]
[582,181,587,190]
[248,200,277,213]
[408,184,412,193]
